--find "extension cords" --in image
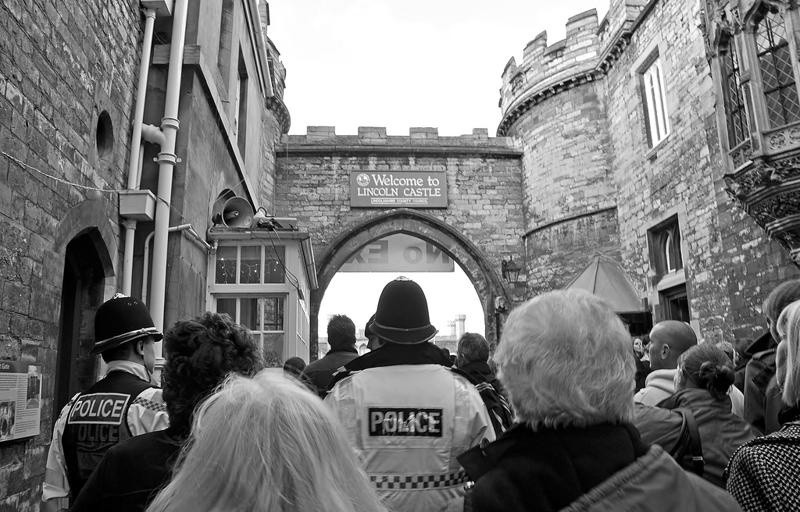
[253,217,298,231]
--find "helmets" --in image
[90,296,164,355]
[370,276,437,346]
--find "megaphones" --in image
[216,197,253,232]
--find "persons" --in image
[631,270,800,511]
[448,333,517,442]
[146,369,388,512]
[299,314,365,398]
[456,286,743,511]
[41,293,169,503]
[283,357,306,378]
[323,275,497,511]
[64,312,265,512]
